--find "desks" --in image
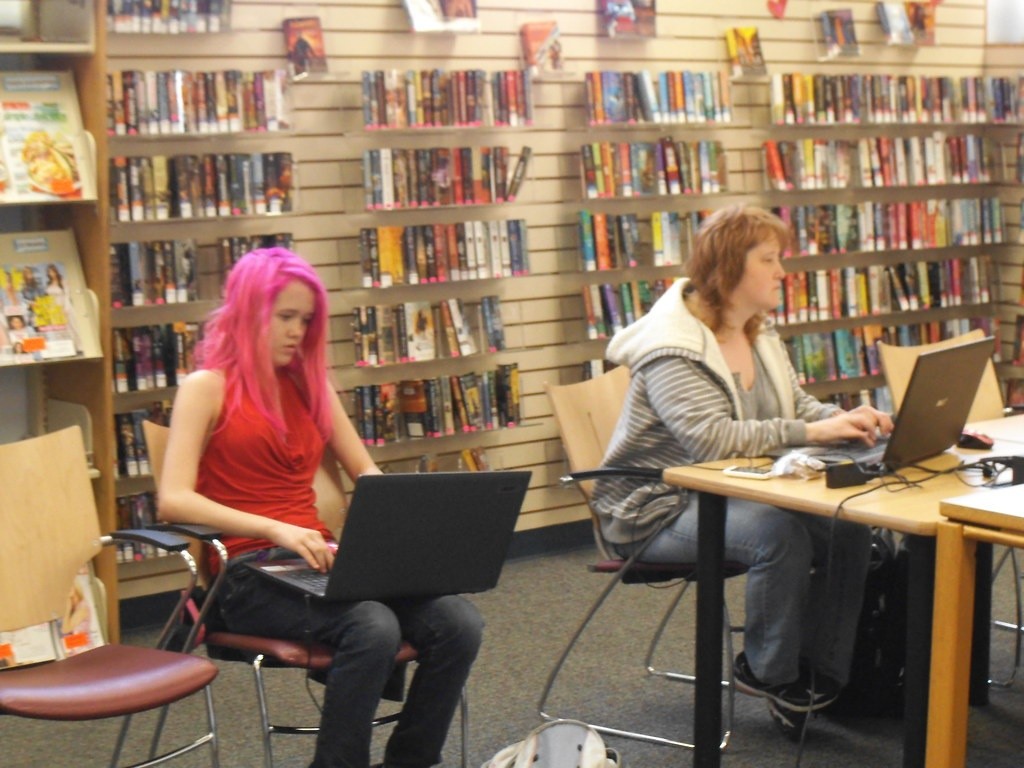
[924,518,1024,768]
[662,430,1024,767]
[962,413,1024,444]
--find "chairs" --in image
[875,327,1024,688]
[141,417,472,768]
[539,363,773,752]
[0,425,219,767]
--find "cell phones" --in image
[723,466,773,480]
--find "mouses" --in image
[956,431,993,449]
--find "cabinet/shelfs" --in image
[359,69,524,445]
[103,0,298,563]
[760,70,1016,409]
[0,0,121,654]
[576,68,736,385]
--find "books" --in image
[0,566,106,678]
[107,3,295,563]
[764,74,1024,436]
[596,1,657,42]
[726,22,766,78]
[523,19,561,70]
[883,2,940,49]
[1,230,104,368]
[817,6,861,57]
[402,0,481,36]
[576,74,730,369]
[0,71,102,205]
[351,69,535,470]
[284,15,328,75]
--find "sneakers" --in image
[732,651,838,711]
[764,694,807,743]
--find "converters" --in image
[825,462,867,488]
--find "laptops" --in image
[240,470,532,604]
[766,336,996,475]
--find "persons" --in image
[589,205,894,733]
[45,265,86,352]
[21,265,46,300]
[9,312,34,340]
[60,579,95,657]
[157,247,486,767]
